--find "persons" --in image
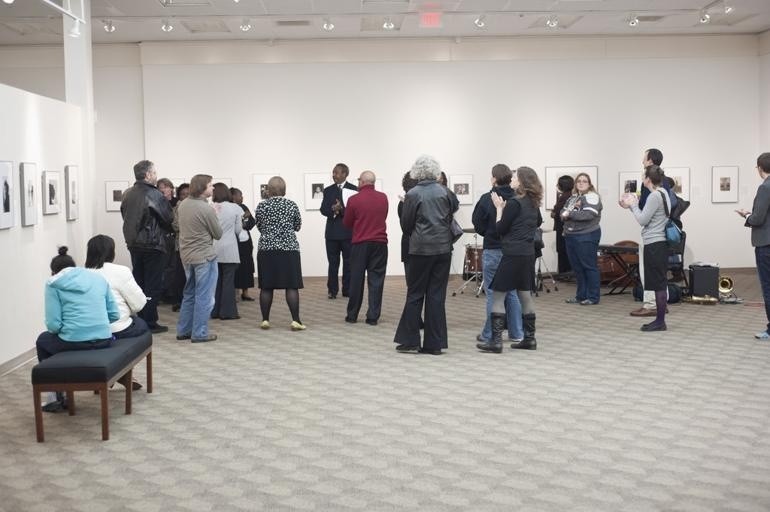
[397,171,424,329]
[228,187,256,302]
[255,176,309,331]
[735,152,770,341]
[172,182,189,310]
[471,163,523,343]
[210,182,245,320]
[174,174,225,343]
[551,175,575,282]
[392,155,460,356]
[343,170,389,326]
[155,179,175,206]
[559,173,603,306]
[320,163,360,301]
[476,166,545,354]
[617,148,689,319]
[35,245,119,413]
[120,160,175,334]
[83,233,151,391]
[621,166,687,333]
[311,183,326,199]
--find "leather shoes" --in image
[240,291,256,302]
[221,315,243,321]
[328,292,338,299]
[290,319,308,331]
[212,313,221,317]
[259,318,271,331]
[641,321,667,332]
[149,324,169,334]
[629,304,660,318]
[191,334,219,343]
[176,334,191,340]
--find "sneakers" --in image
[566,294,580,304]
[415,346,444,355]
[580,299,598,305]
[502,333,525,342]
[366,316,376,325]
[754,331,770,339]
[396,344,420,355]
[344,315,357,323]
[477,334,495,342]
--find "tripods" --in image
[451,231,483,297]
[535,230,559,296]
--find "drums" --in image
[463,249,485,280]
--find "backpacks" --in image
[652,189,683,253]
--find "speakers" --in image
[688,266,719,304]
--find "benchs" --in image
[31,330,153,445]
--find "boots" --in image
[510,312,539,352]
[475,310,506,356]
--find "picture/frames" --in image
[304,173,334,210]
[450,174,473,205]
[65,165,80,220]
[0,160,13,229]
[664,167,690,201]
[712,166,740,202]
[43,170,61,216]
[544,165,598,211]
[253,175,279,206]
[618,171,643,204]
[106,181,129,212]
[169,179,183,196]
[213,180,230,191]
[20,162,38,227]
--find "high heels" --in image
[109,374,143,394]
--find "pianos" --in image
[599,243,641,258]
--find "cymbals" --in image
[462,228,477,234]
